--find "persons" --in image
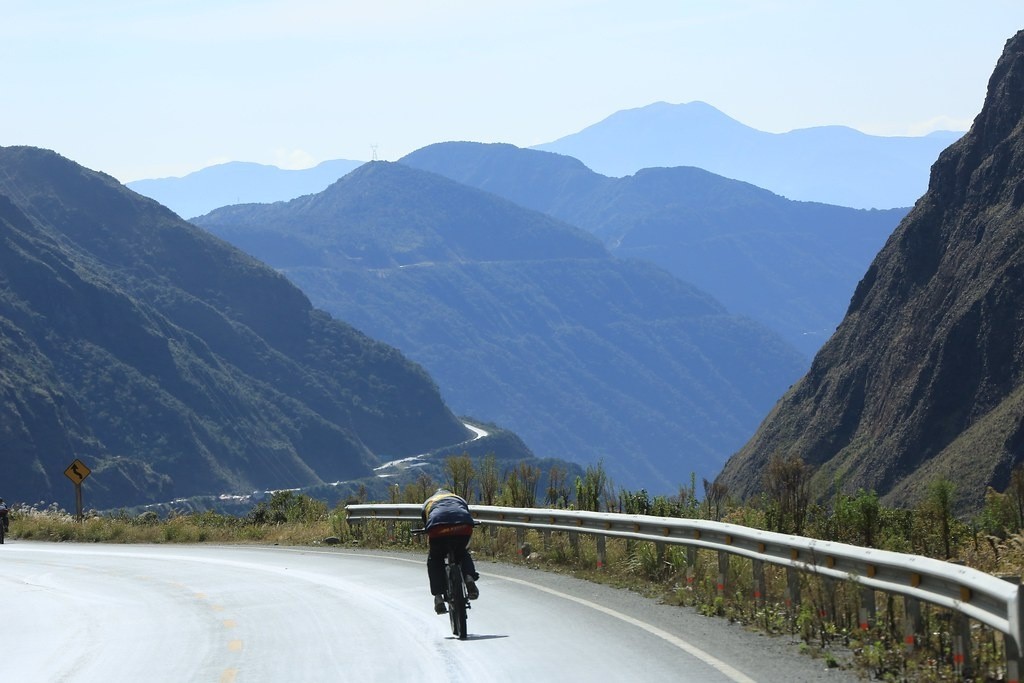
[422,488,480,612]
[0,497,9,532]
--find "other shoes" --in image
[464,575,479,598]
[434,595,446,612]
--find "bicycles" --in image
[410,520,483,640]
